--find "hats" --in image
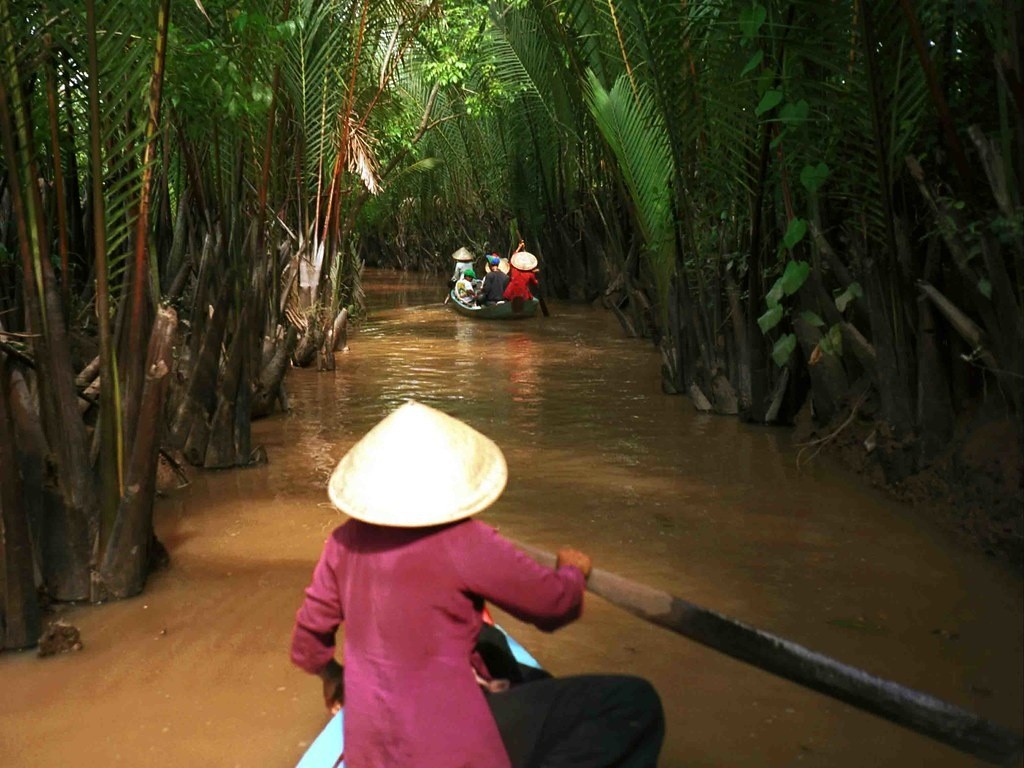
[452,247,473,260]
[510,251,538,271]
[485,257,510,275]
[464,269,478,280]
[326,400,508,529]
[486,255,499,266]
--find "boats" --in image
[450,278,540,318]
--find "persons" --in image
[447,240,539,306]
[291,398,666,768]
